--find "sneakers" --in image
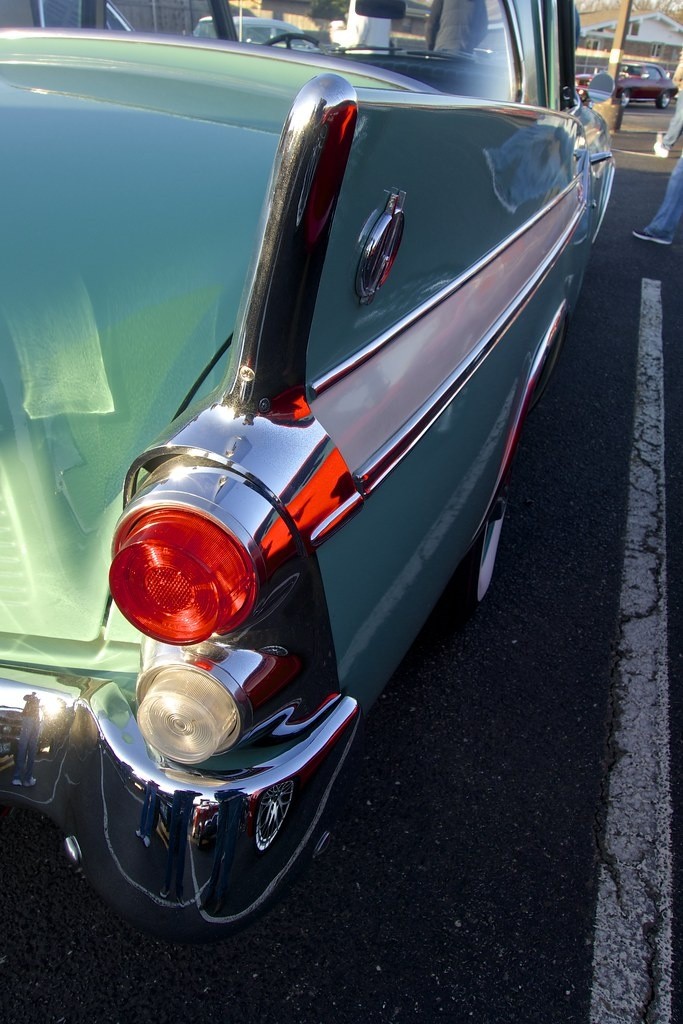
[633,229,672,244]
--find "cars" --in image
[193,17,314,50]
[0,0,616,936]
[575,61,680,109]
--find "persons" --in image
[424,0,488,64]
[631,65,683,244]
[329,0,390,56]
